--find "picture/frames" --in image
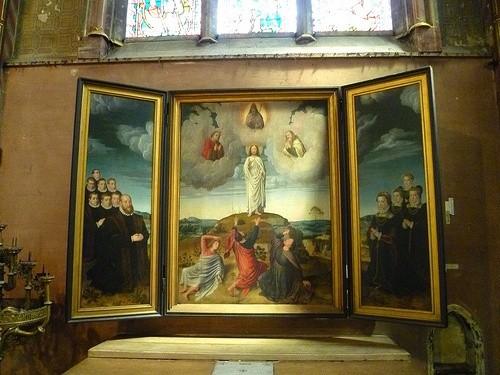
[340,64,446,331]
[163,85,349,320]
[64,78,170,325]
